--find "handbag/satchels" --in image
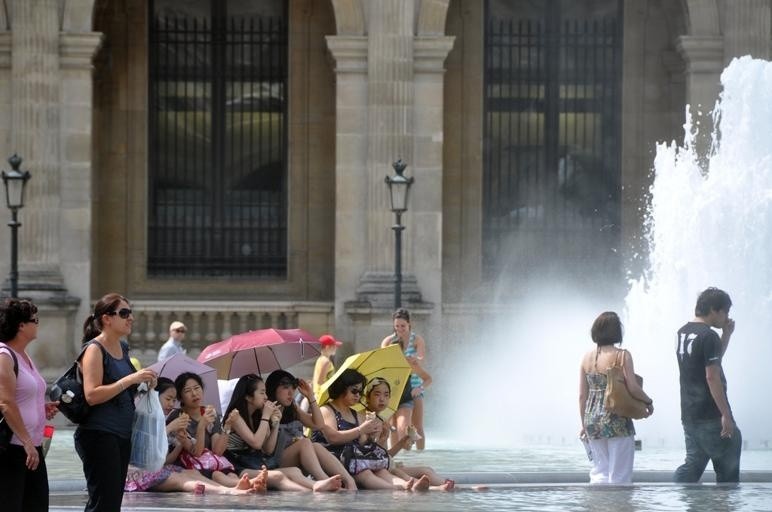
[50,361,92,425]
[603,365,650,419]
[179,448,235,472]
[340,441,390,474]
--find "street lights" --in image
[384,159,414,315]
[1,154,29,299]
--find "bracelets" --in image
[259,417,270,423]
[356,426,362,437]
[22,437,35,446]
[117,378,127,391]
[417,384,425,393]
[644,398,654,406]
[309,399,317,405]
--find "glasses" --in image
[110,308,131,319]
[350,388,364,396]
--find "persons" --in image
[668,284,743,484]
[165,371,270,494]
[385,334,432,448]
[74,294,160,512]
[357,376,457,493]
[576,309,657,487]
[221,372,342,496]
[1,298,64,511]
[311,333,343,395]
[252,369,360,491]
[123,376,256,498]
[308,368,431,491]
[378,307,432,454]
[157,319,189,366]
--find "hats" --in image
[169,321,187,331]
[319,334,342,348]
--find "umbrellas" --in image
[195,327,323,381]
[133,352,224,423]
[302,343,412,439]
